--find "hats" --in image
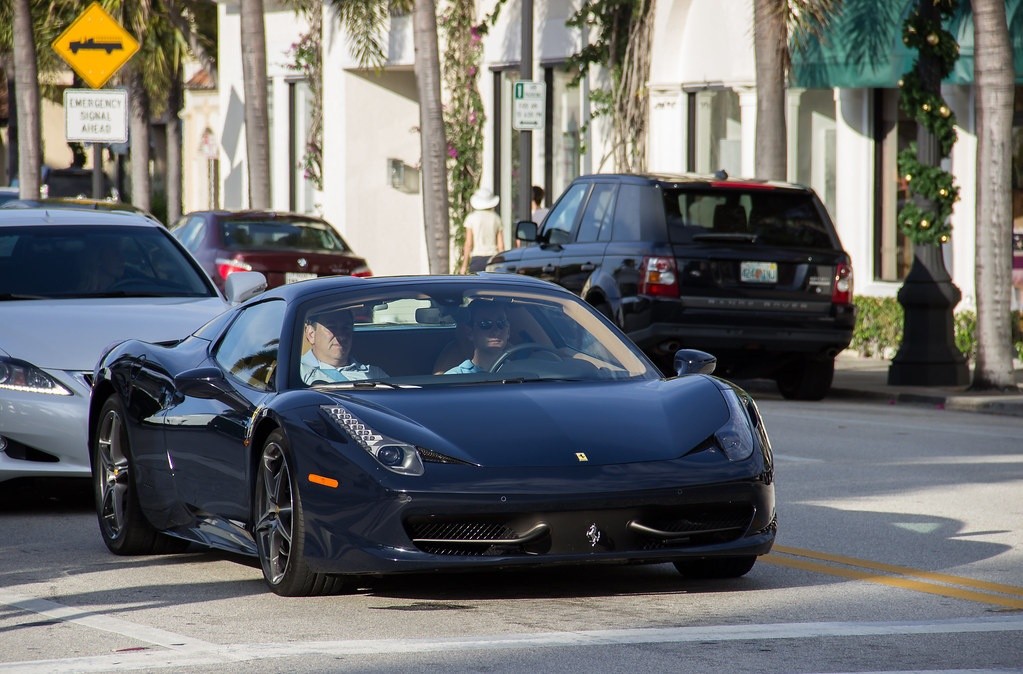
[470,188,499,209]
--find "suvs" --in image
[482,172,857,400]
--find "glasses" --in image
[478,319,505,330]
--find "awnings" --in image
[788,0,1023,88]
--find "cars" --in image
[0,166,170,214]
[0,206,268,482]
[164,209,375,325]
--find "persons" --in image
[531,185,550,229]
[433,299,514,375]
[442,298,513,374]
[459,189,504,276]
[80,234,137,294]
[299,309,391,386]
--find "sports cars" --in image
[84,271,777,598]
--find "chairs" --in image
[712,204,747,234]
